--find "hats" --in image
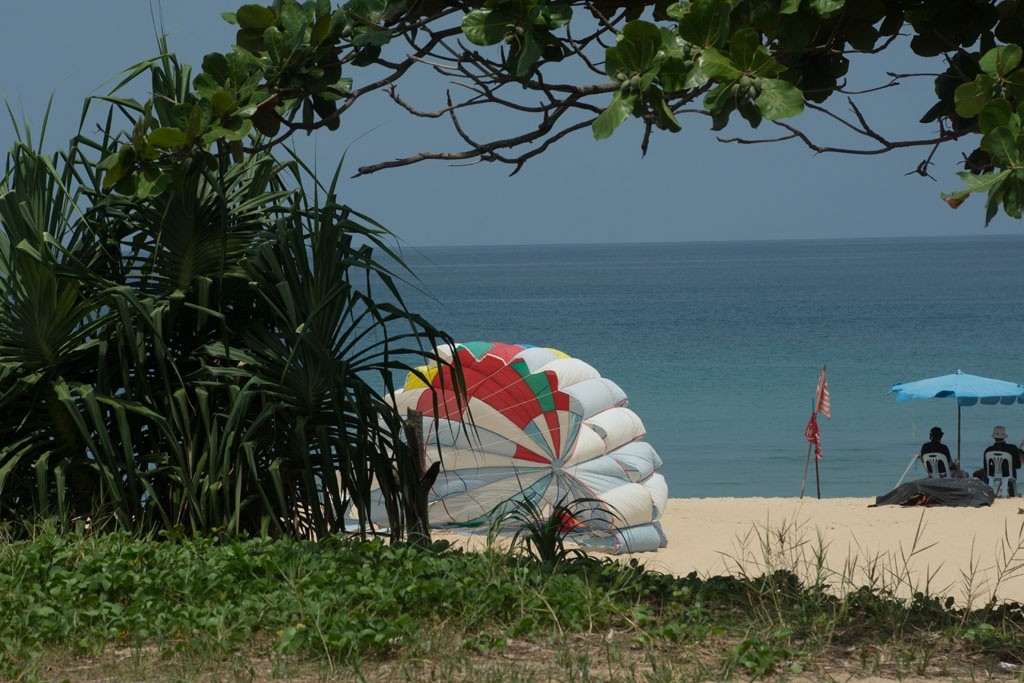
[930,427,945,437]
[991,426,1008,438]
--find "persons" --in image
[920,425,962,479]
[971,425,1021,496]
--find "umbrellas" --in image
[886,368,1024,478]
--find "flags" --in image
[817,369,831,420]
[804,412,823,460]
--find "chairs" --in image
[922,453,954,479]
[985,451,1017,498]
